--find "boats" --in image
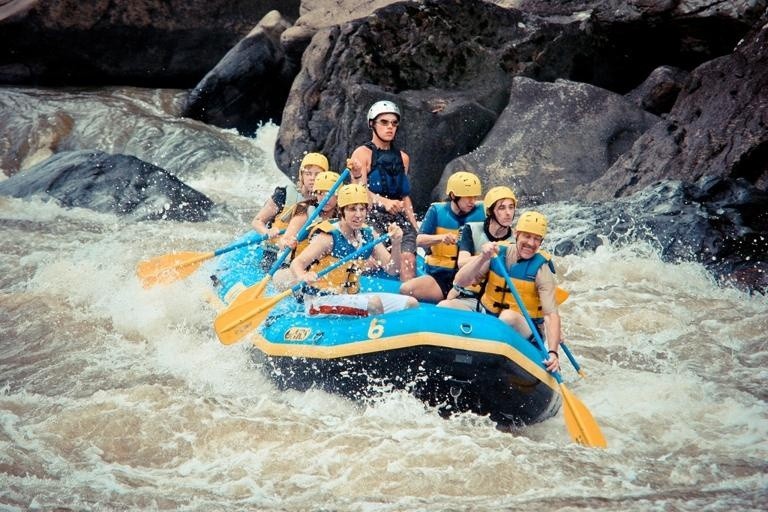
[213,222,564,424]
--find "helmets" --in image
[516,211,547,239]
[337,183,369,208]
[483,186,517,212]
[367,100,401,129]
[300,153,329,171]
[313,171,343,193]
[445,172,481,197]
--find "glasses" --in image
[374,119,399,127]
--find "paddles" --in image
[226,158,353,310]
[136,229,286,289]
[215,227,399,345]
[492,251,608,449]
[455,240,569,305]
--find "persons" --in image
[290,184,419,317]
[251,153,330,278]
[350,100,419,283]
[416,171,486,275]
[273,159,364,305]
[399,186,517,304]
[436,211,561,373]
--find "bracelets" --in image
[548,351,558,359]
[354,175,362,179]
